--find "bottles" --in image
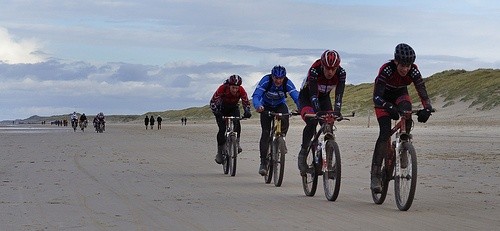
[317,134,324,151]
[390,141,397,163]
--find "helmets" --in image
[320,49,341,69]
[271,66,285,78]
[229,75,243,86]
[395,43,416,65]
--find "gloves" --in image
[216,112,223,119]
[243,112,252,118]
[314,108,326,120]
[333,108,343,121]
[385,103,401,121]
[416,108,431,123]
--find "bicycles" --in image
[371,108,436,212]
[217,117,246,176]
[301,111,355,202]
[259,107,300,187]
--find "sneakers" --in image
[400,148,410,169]
[257,160,269,176]
[298,157,310,175]
[371,175,384,192]
[215,152,224,163]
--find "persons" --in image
[296,48,346,181]
[369,42,433,193]
[251,65,302,175]
[208,73,254,164]
[42,110,188,133]
